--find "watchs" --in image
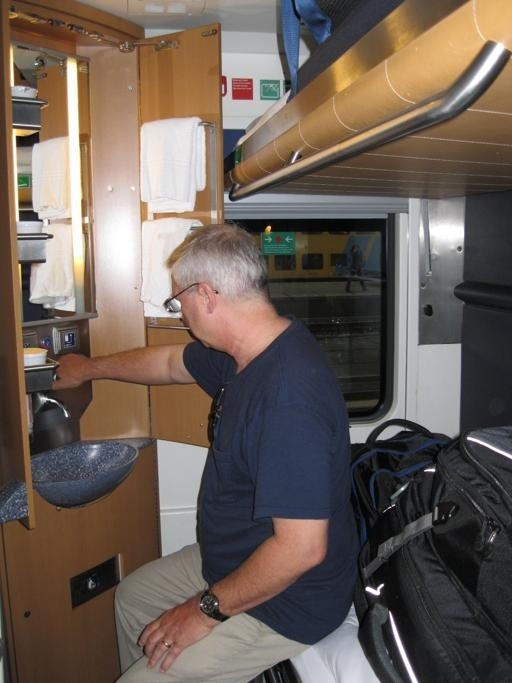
[199,588,231,623]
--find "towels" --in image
[139,116,206,215]
[139,220,208,319]
[30,136,77,219]
[30,226,85,314]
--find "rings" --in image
[160,641,171,648]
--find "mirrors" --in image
[10,44,96,328]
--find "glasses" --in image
[163,282,220,313]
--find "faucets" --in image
[33,392,72,420]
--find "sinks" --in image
[31,442,139,506]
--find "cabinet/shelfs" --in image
[1,1,225,682]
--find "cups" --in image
[23,347,48,366]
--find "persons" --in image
[52,223,361,682]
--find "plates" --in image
[9,85,41,98]
